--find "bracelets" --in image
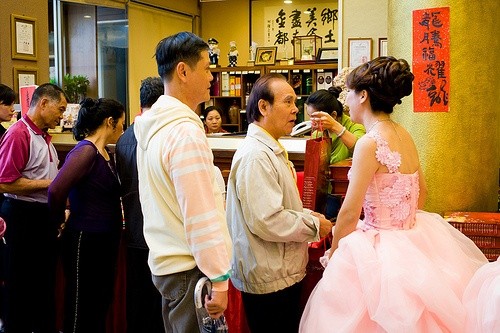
[338,126,346,137]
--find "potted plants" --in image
[63,73,90,104]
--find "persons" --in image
[133,31,234,333]
[208,37,220,66]
[115,76,164,333]
[299,56,500,333]
[307,86,366,193]
[0,83,70,333]
[0,83,17,140]
[47,97,125,333]
[223,72,333,333]
[227,41,238,68]
[203,106,228,133]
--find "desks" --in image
[50,133,306,166]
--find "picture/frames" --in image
[254,47,277,66]
[315,48,338,63]
[249,0,338,62]
[13,67,40,102]
[10,14,38,62]
[379,38,387,57]
[348,38,372,67]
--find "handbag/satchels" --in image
[301,111,332,213]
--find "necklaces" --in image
[367,118,391,134]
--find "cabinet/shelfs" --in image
[195,64,338,132]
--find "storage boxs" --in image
[293,35,322,65]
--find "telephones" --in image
[290,121,312,137]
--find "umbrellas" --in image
[194,276,230,333]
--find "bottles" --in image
[296,96,304,125]
[228,100,239,124]
[245,82,253,110]
[293,74,312,95]
